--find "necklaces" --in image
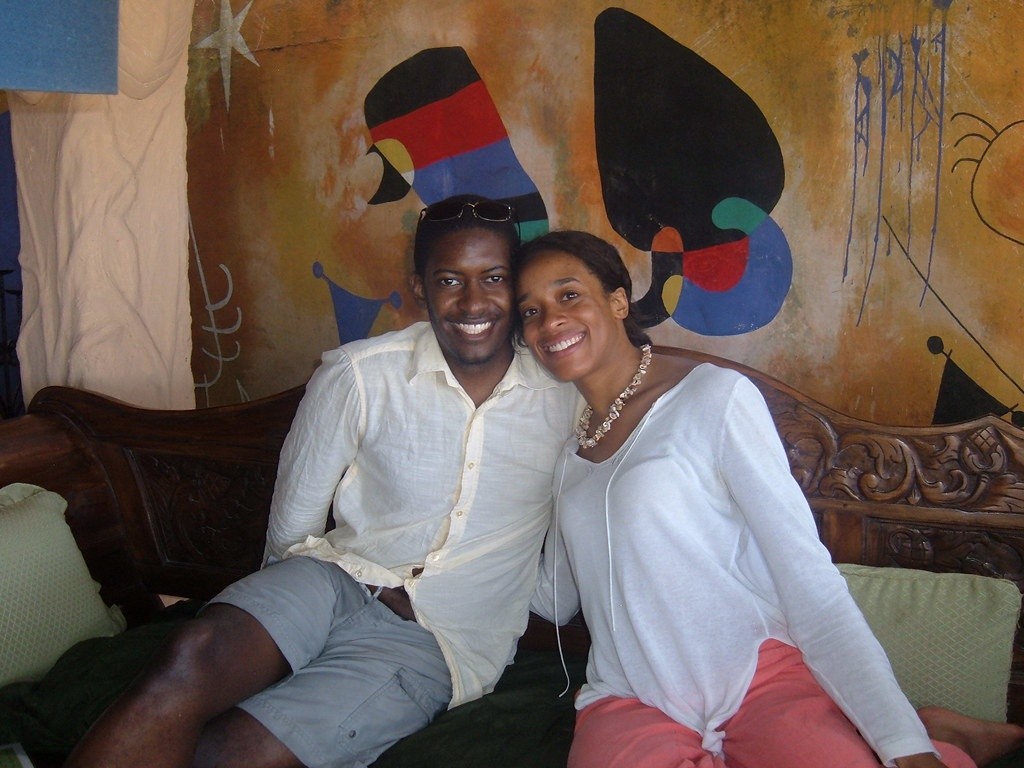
[574,344,652,450]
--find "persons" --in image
[512,228,1024,768]
[61,193,587,768]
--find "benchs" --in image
[0,343,1024,768]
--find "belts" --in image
[365,583,417,622]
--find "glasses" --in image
[416,200,521,247]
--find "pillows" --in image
[0,481,125,692]
[834,563,1021,724]
